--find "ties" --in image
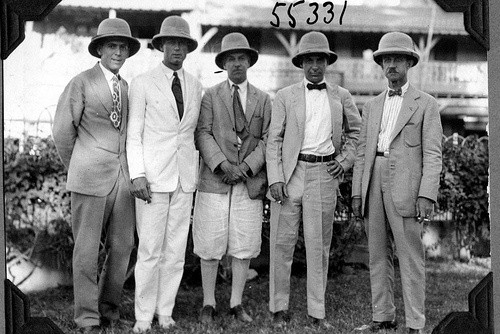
[171,72,184,122]
[109,76,122,128]
[232,84,250,140]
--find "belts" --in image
[298,153,336,163]
[376,151,390,158]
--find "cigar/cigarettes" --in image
[277,201,282,204]
[145,200,147,205]
[417,217,430,222]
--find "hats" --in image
[151,14,198,53]
[88,17,141,58]
[215,31,258,71]
[291,30,337,68]
[372,31,420,68]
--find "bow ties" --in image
[388,88,402,97]
[306,83,327,90]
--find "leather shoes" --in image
[197,305,216,326]
[132,319,152,334]
[159,315,176,330]
[353,319,397,334]
[101,315,123,334]
[271,311,287,331]
[227,304,254,324]
[80,325,104,334]
[305,316,335,333]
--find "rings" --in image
[427,214,430,217]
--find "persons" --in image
[352,31,443,333]
[190,32,274,328]
[265,31,365,329]
[51,18,137,334]
[125,15,207,333]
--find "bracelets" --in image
[131,173,147,182]
